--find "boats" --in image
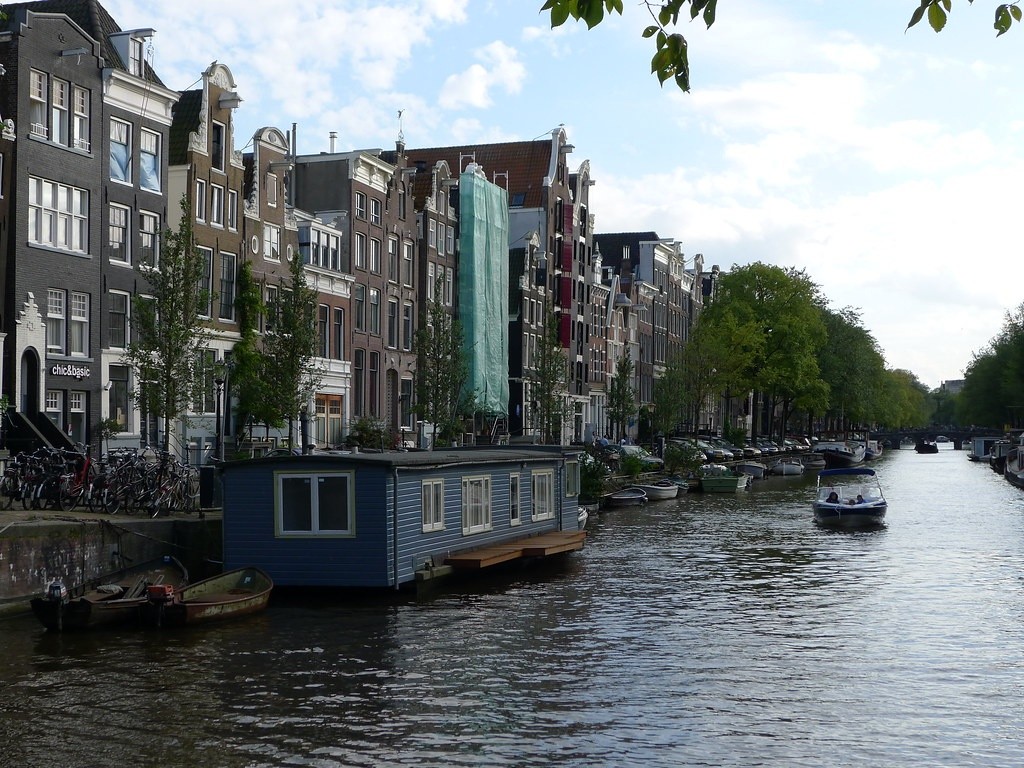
[730,451,826,478]
[634,477,690,502]
[30,551,188,634]
[697,471,748,493]
[969,433,1024,489]
[600,487,649,509]
[813,439,883,464]
[811,467,887,528]
[916,442,939,453]
[577,503,589,530]
[147,565,274,626]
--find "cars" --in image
[602,443,665,474]
[669,434,818,463]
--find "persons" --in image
[826,492,839,504]
[592,431,626,445]
[856,495,866,504]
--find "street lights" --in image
[209,356,230,463]
[708,410,714,443]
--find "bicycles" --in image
[0,440,200,521]
